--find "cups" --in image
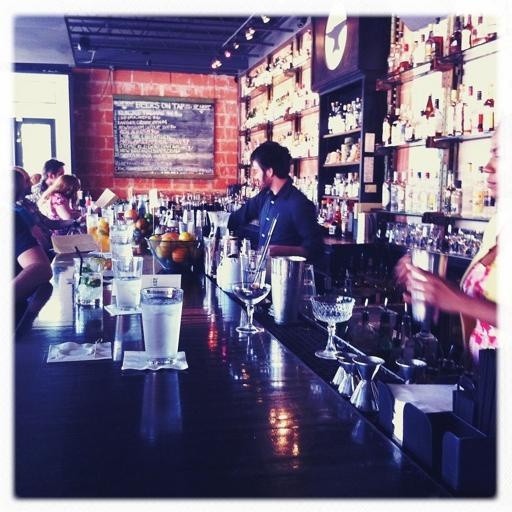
[96,216,110,253]
[140,286,185,366]
[112,255,144,310]
[72,257,106,306]
[271,255,307,326]
[394,359,427,381]
[110,224,133,259]
[87,213,98,243]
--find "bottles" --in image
[238,28,315,203]
[317,95,365,242]
[107,191,240,238]
[380,11,495,257]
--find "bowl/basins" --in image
[145,236,201,273]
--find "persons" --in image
[40,158,65,193]
[227,141,324,259]
[14,166,102,232]
[14,169,50,252]
[13,210,53,300]
[35,174,80,227]
[31,174,42,184]
[394,123,499,370]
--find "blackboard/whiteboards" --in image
[113,94,216,180]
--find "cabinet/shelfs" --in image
[236,14,495,260]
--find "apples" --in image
[123,209,139,220]
[149,231,203,262]
[136,217,147,229]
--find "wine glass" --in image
[309,295,355,361]
[407,245,450,344]
[329,350,386,411]
[232,282,271,334]
